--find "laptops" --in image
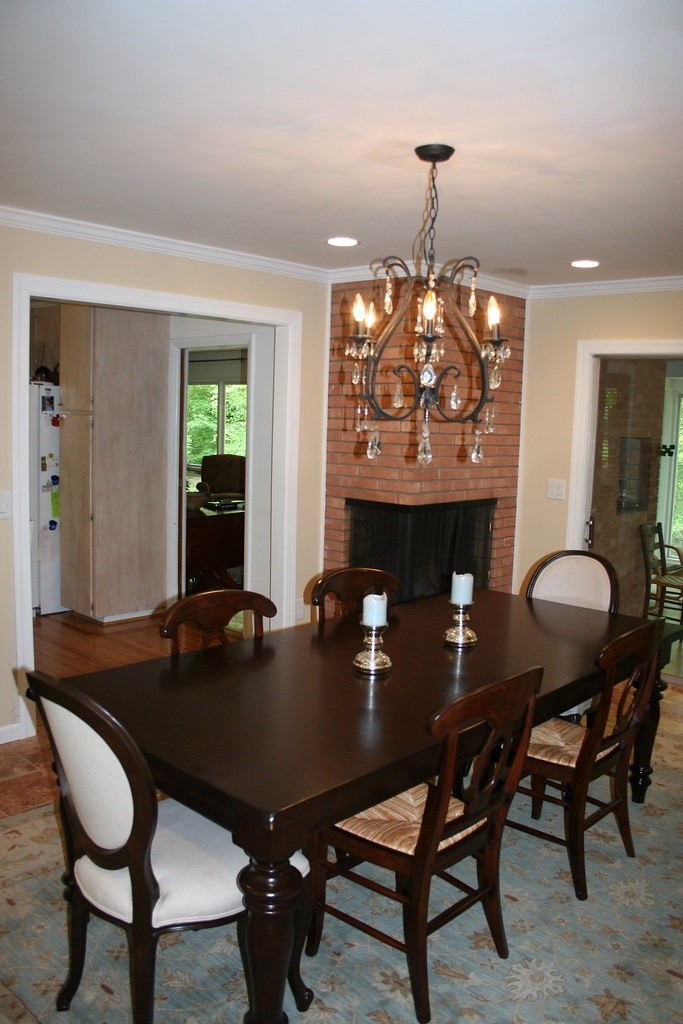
[207,500,245,508]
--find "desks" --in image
[62,587,683,1024]
[186,492,244,587]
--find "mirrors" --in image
[616,436,651,513]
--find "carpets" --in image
[0,754,683,1024]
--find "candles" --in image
[451,571,474,605]
[362,591,388,627]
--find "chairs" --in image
[24,671,317,1024]
[196,454,245,495]
[303,567,397,621]
[291,666,546,1024]
[638,520,683,644]
[472,617,667,897]
[526,549,620,724]
[158,590,277,654]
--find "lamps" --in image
[329,142,509,466]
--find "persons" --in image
[45,400,53,411]
